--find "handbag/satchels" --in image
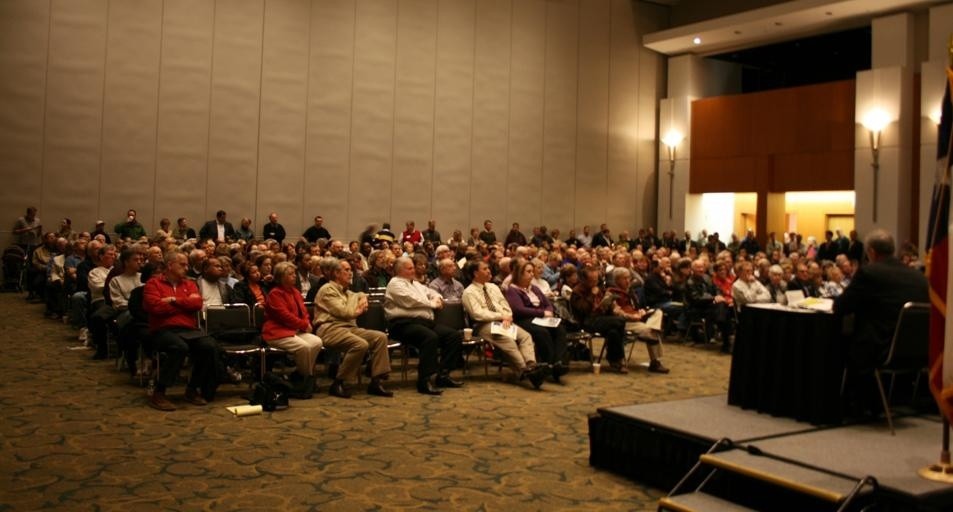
[250,378,289,411]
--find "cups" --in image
[592,363,601,375]
[464,328,473,341]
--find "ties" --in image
[483,288,496,311]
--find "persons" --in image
[592,224,863,343]
[15,207,42,265]
[833,229,929,418]
[384,256,465,395]
[568,267,629,374]
[263,263,323,394]
[141,251,208,410]
[314,259,393,396]
[608,266,669,374]
[32,206,596,388]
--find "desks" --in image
[727,303,904,427]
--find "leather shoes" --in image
[149,395,176,410]
[649,360,669,373]
[329,383,350,397]
[368,384,393,397]
[417,378,462,394]
[523,363,553,388]
[638,329,658,344]
[185,388,207,405]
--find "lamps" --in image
[668,144,676,163]
[869,129,882,153]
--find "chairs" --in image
[838,302,931,436]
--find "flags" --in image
[925,66,953,423]
